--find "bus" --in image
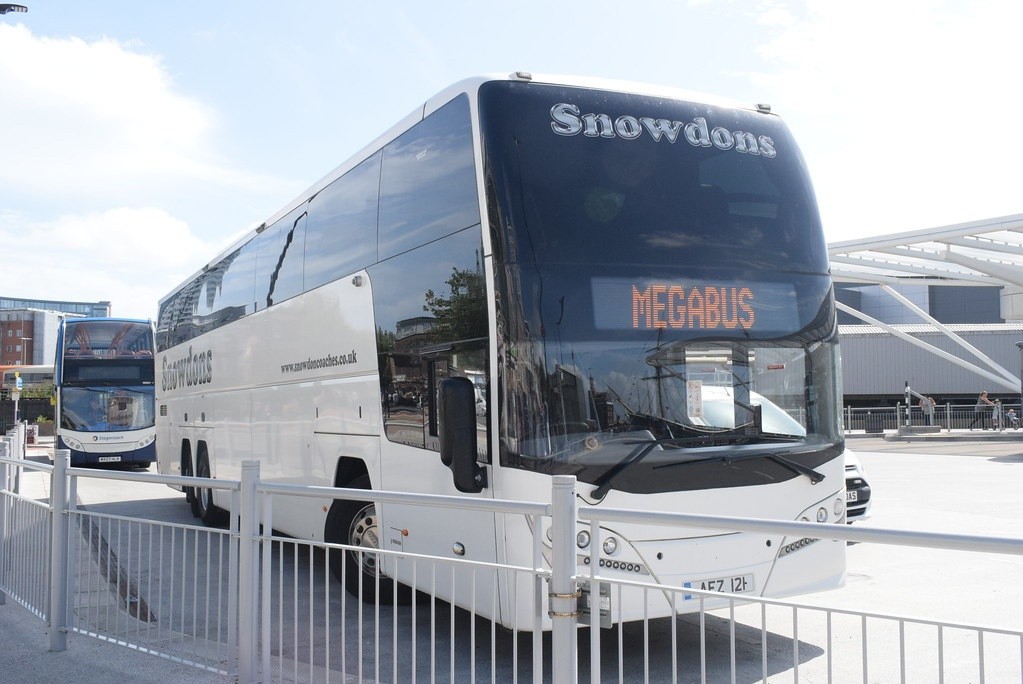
[50,315,157,471]
[149,69,848,634]
[0,364,54,400]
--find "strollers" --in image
[998,406,1020,430]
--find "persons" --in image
[992,398,999,428]
[969,391,995,430]
[918,394,937,426]
[88,402,106,421]
[1006,407,1018,428]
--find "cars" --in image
[687,383,872,526]
